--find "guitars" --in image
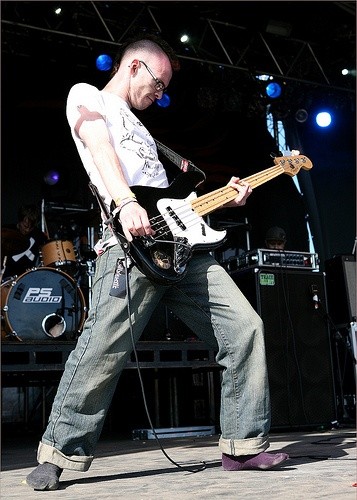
[109,150,314,286]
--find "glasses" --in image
[139,60,169,95]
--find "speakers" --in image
[227,267,336,434]
[325,254,357,324]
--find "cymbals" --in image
[86,209,102,228]
[1,225,31,256]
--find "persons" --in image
[1,208,45,280]
[266,226,287,251]
[26,33,290,491]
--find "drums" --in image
[0,267,87,341]
[41,239,77,269]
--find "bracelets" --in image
[112,199,139,218]
[115,193,136,206]
